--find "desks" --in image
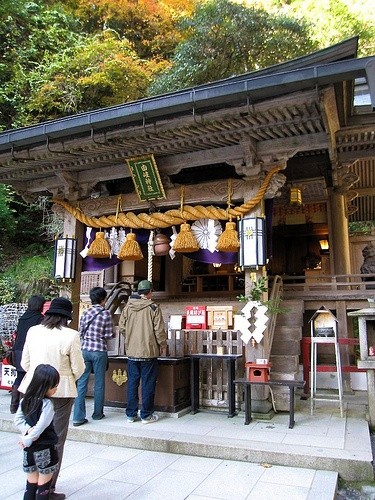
[183,353,243,418]
[189,273,240,293]
[104,355,192,413]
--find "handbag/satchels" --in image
[90,357,109,374]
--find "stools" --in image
[233,378,306,429]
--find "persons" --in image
[72,287,116,426]
[18,297,86,493]
[119,280,168,424]
[10,293,47,414]
[14,364,66,500]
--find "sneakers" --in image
[128,414,141,423]
[142,415,158,423]
[73,419,88,426]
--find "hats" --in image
[138,279,152,290]
[44,297,72,320]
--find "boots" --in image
[10,386,20,414]
[23,480,37,500]
[37,479,65,500]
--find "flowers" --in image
[4,331,17,347]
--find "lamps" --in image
[53,235,78,283]
[237,214,266,272]
[286,183,302,207]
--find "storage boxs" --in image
[206,305,238,329]
[186,306,209,329]
